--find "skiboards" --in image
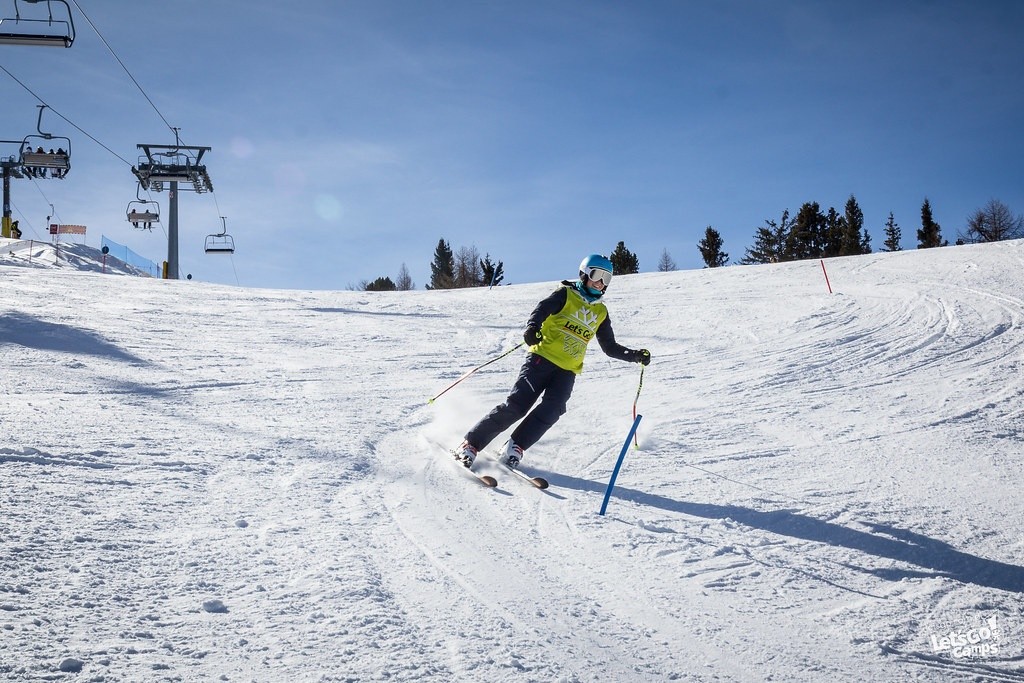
[423,430,498,488]
[478,448,549,489]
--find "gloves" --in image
[636,349,651,366]
[523,325,543,346]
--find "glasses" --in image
[588,268,613,285]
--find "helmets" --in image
[578,254,613,282]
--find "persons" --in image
[11,220,22,239]
[21,146,72,179]
[131,209,138,228]
[456,254,651,469]
[144,209,151,229]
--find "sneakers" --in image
[499,437,523,468]
[455,441,477,468]
